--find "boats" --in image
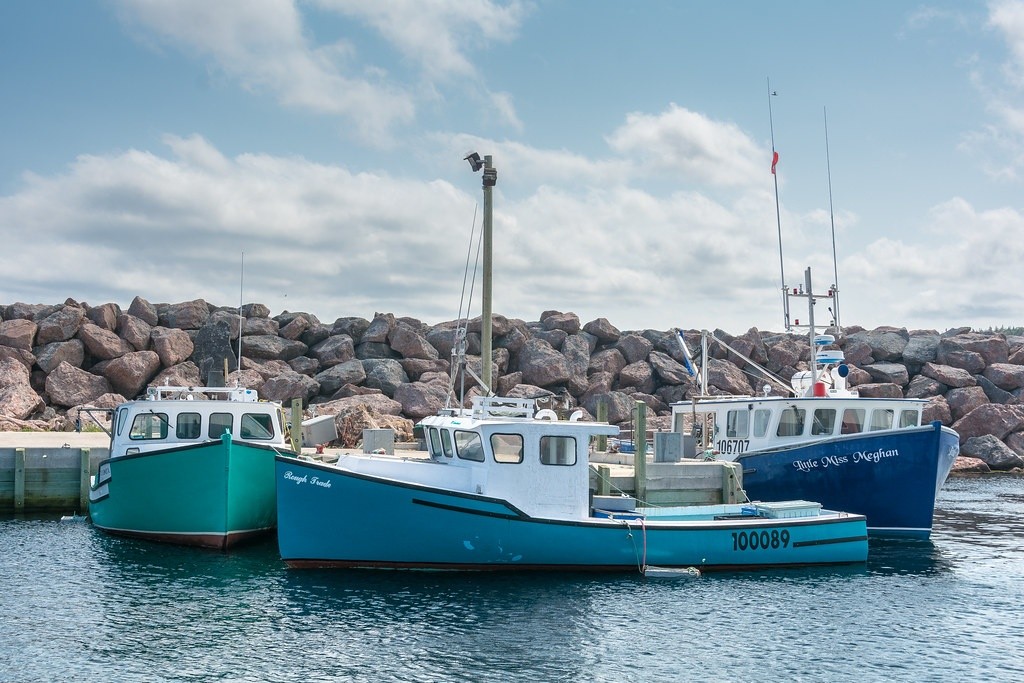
[88,251,299,551]
[615,74,959,547]
[275,147,870,572]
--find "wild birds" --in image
[771,91,779,96]
